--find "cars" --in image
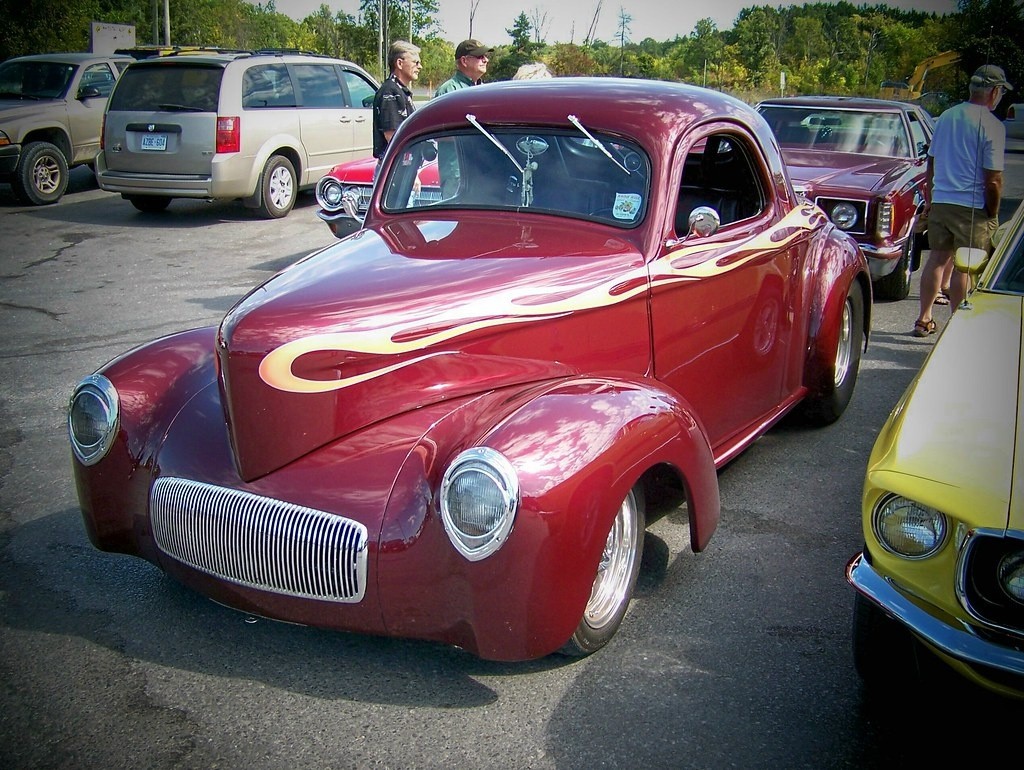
[312,104,733,242]
[732,92,935,304]
[845,195,1024,716]
[65,70,877,668]
[1000,103,1023,141]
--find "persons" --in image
[513,64,551,80]
[373,40,422,208]
[914,65,1013,336]
[433,40,495,200]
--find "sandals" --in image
[934,288,950,305]
[913,318,937,336]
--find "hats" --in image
[973,65,1014,89]
[455,39,495,60]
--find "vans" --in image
[95,44,383,222]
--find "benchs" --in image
[531,177,758,237]
[780,141,903,154]
[808,129,903,145]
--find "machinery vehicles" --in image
[878,46,969,101]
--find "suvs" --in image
[0,52,139,207]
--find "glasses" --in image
[465,53,487,59]
[995,86,1008,95]
[406,58,423,66]
